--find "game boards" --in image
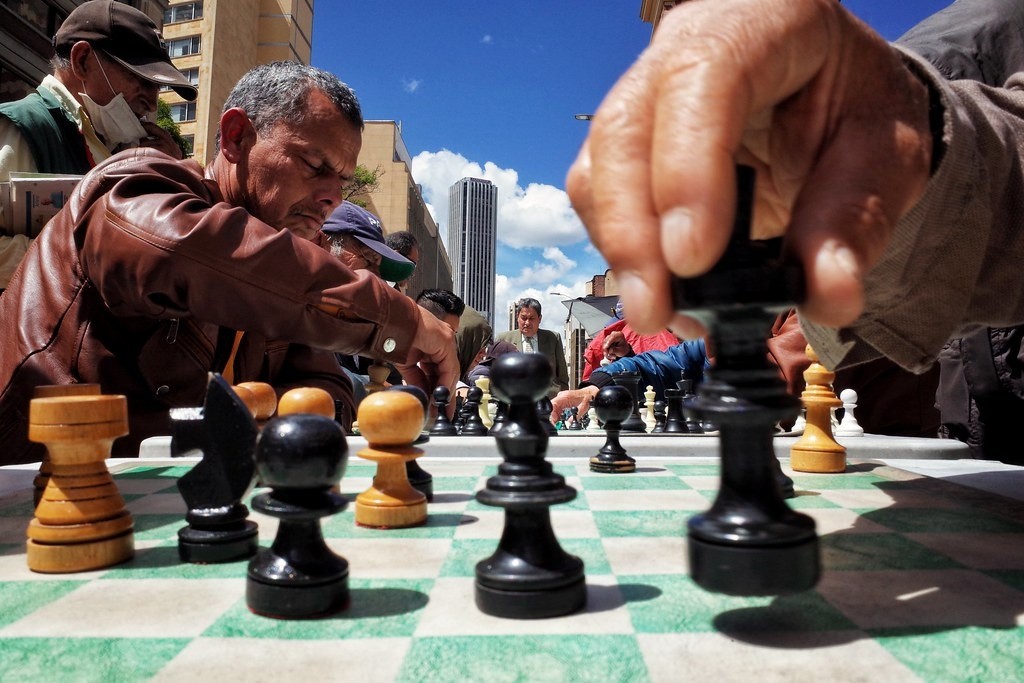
[0,451,1024,683]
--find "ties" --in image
[525,338,534,353]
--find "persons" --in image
[318,200,418,426]
[601,330,636,361]
[562,1,1024,377]
[413,289,466,340]
[381,230,420,293]
[1,58,461,468]
[548,333,716,425]
[469,340,520,387]
[453,304,492,382]
[0,0,199,298]
[492,298,570,394]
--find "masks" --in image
[80,51,147,144]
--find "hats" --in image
[602,300,624,326]
[321,200,415,283]
[50,0,198,101]
